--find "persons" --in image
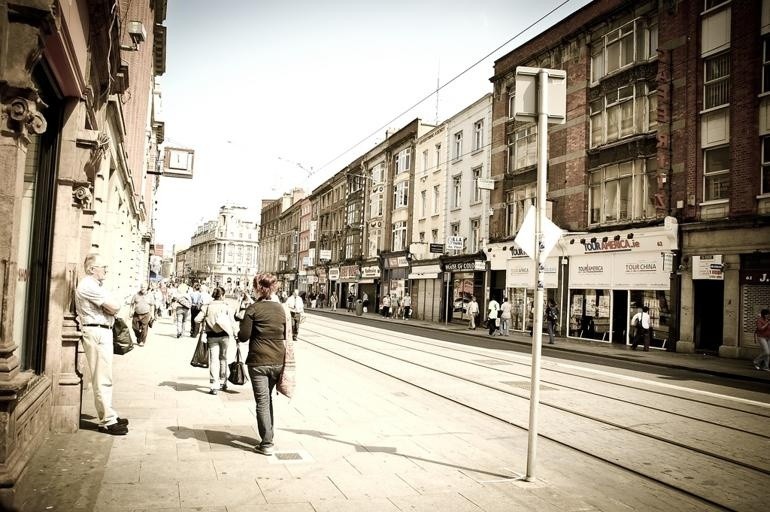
[545,299,561,344]
[129,270,413,395]
[500,296,513,337]
[632,306,652,352]
[486,295,500,336]
[753,307,770,371]
[233,273,290,455]
[72,253,129,436]
[468,293,479,329]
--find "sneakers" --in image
[209,389,217,395]
[221,384,226,390]
[254,444,273,455]
[97,418,128,434]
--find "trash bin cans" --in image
[356,299,363,316]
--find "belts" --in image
[83,324,112,329]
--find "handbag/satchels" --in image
[276,351,295,398]
[148,317,155,328]
[178,297,192,308]
[191,341,209,368]
[113,318,134,355]
[228,362,249,385]
